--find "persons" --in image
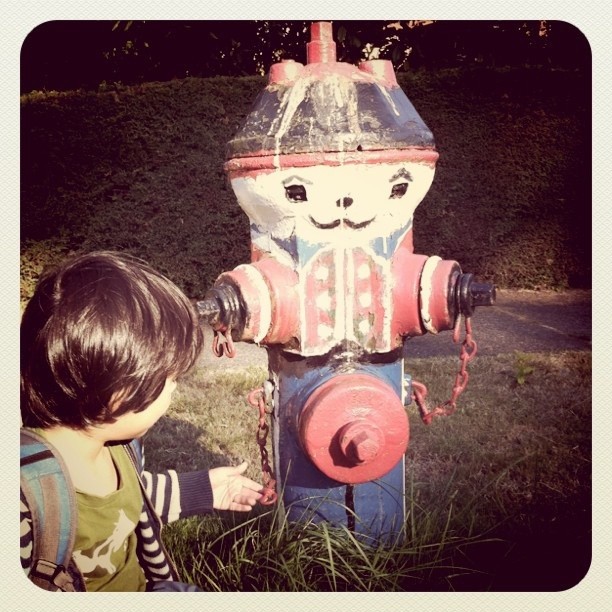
[20,250,265,591]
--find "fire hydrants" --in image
[197,21,495,550]
